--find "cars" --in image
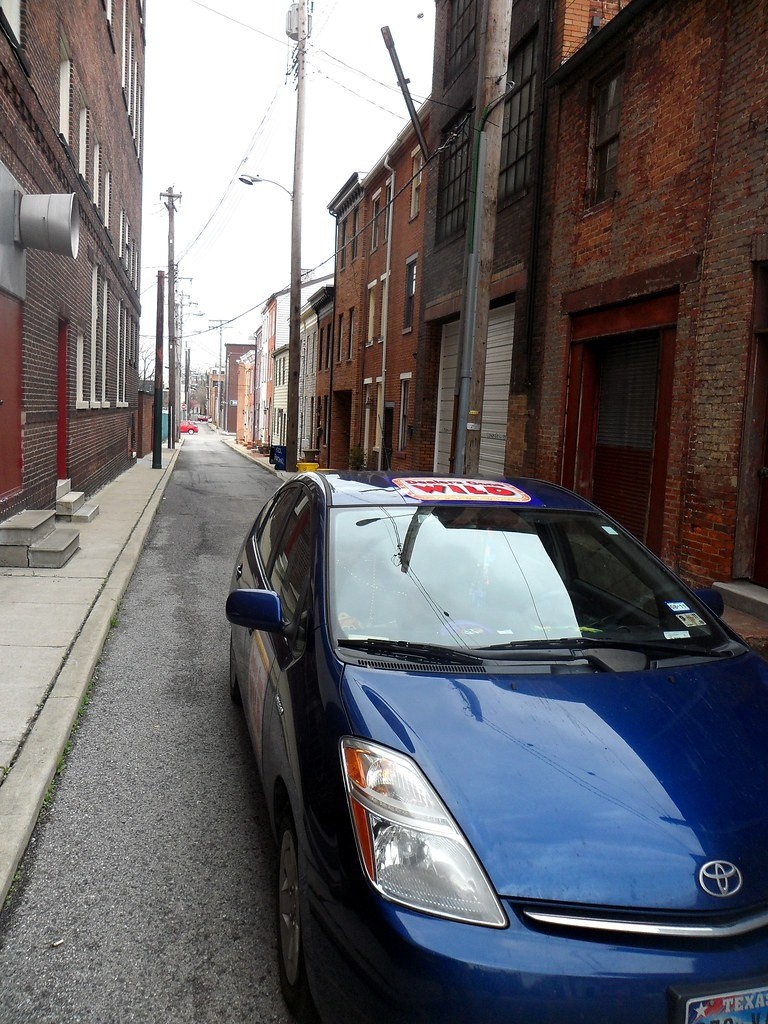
[198,415,211,423]
[225,471,768,1024]
[180,420,198,435]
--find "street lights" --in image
[237,174,303,471]
[173,312,204,444]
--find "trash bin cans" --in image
[273,446,286,469]
